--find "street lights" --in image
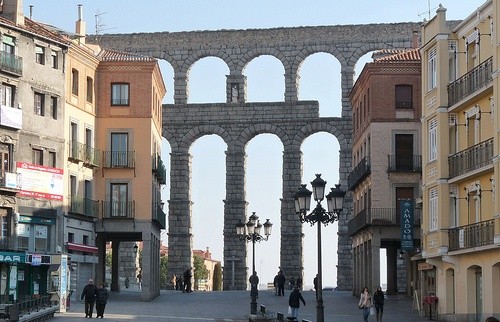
[293,173,346,322]
[234,211,274,315]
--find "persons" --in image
[373,285,384,322]
[125,276,130,288]
[249,271,259,299]
[314,274,319,301]
[95,281,110,319]
[359,287,373,322]
[169,273,184,291]
[273,270,285,297]
[296,275,303,291]
[288,276,295,291]
[80,278,98,318]
[182,267,194,293]
[288,285,306,322]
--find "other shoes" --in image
[85,316,88,318]
[96,315,99,318]
[101,315,103,318]
[89,315,92,318]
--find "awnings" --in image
[0,248,25,264]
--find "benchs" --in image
[5,295,58,322]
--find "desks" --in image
[422,295,438,321]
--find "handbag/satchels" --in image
[359,303,364,309]
[369,307,376,317]
[288,305,293,315]
